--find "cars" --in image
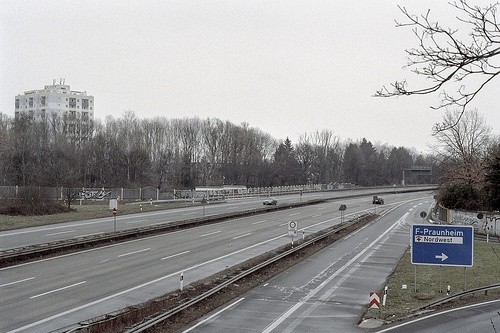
[373,197,384,205]
[263,197,278,205]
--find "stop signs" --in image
[112,207,118,216]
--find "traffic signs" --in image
[411,224,474,267]
